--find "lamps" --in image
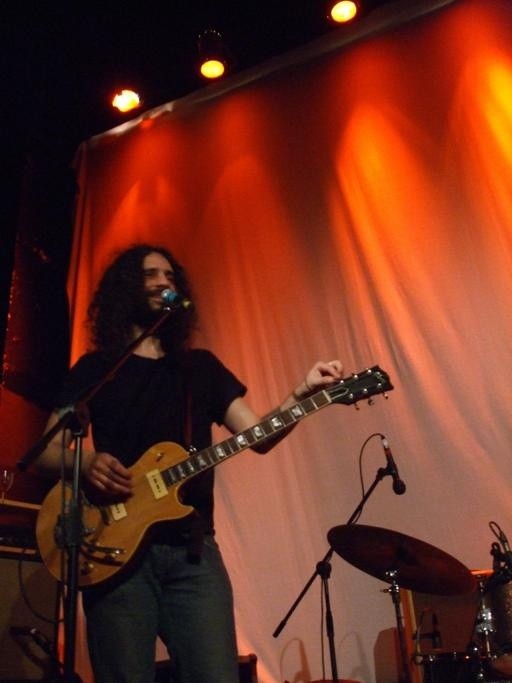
[194,30,231,82]
[326,0,362,28]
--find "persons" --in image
[35,243,344,683]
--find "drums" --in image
[410,566,512,662]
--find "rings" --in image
[329,360,333,366]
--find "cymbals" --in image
[327,524,478,597]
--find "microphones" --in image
[161,289,195,312]
[379,436,407,495]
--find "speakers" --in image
[399,568,512,683]
[0,498,65,683]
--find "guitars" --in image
[35,364,394,590]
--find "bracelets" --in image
[305,377,312,391]
[292,388,302,401]
